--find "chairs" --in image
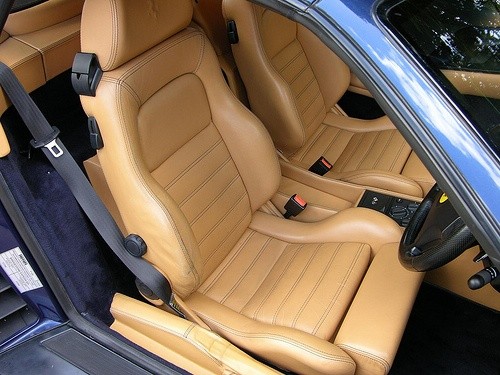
[0,0,438,375]
